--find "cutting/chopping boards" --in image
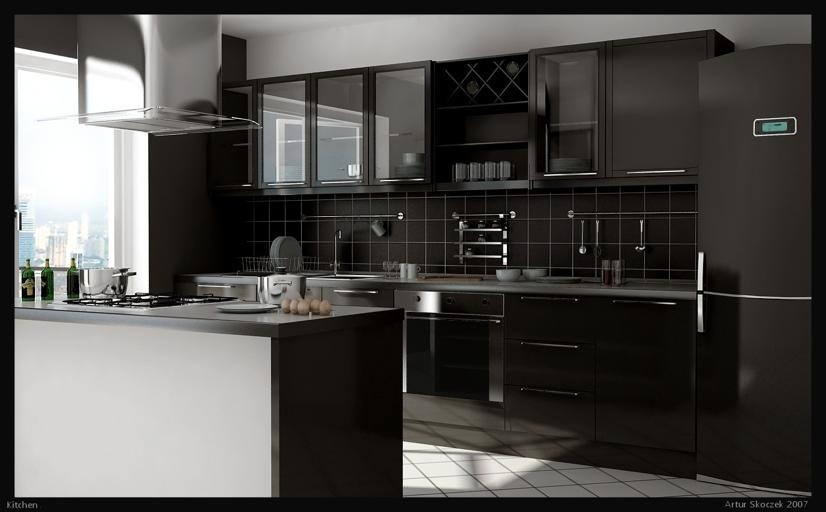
[416,276,483,282]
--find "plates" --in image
[270,236,304,272]
[394,153,424,178]
[551,158,593,173]
[535,276,582,284]
[278,165,304,181]
[216,303,280,313]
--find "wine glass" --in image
[383,261,400,279]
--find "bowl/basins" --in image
[523,268,548,280]
[495,269,521,282]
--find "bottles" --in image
[465,247,473,255]
[40,259,54,300]
[21,258,36,301]
[478,220,485,229]
[21,301,35,309]
[492,220,499,228]
[66,258,79,298]
[40,301,53,309]
[462,220,471,229]
[477,234,485,242]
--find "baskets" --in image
[242,256,319,272]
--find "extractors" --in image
[34,14,263,138]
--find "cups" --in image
[482,160,500,180]
[400,263,410,279]
[467,161,484,180]
[497,160,516,181]
[408,264,421,280]
[610,260,627,286]
[370,220,387,239]
[602,260,612,285]
[452,163,467,181]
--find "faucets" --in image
[329,229,342,274]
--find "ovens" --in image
[392,289,505,403]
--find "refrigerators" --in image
[695,44,822,497]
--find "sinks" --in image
[317,273,383,279]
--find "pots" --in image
[77,267,136,300]
[337,163,362,179]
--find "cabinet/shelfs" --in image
[450,208,518,269]
[180,282,260,302]
[531,40,606,182]
[210,79,255,193]
[321,288,393,308]
[609,30,737,186]
[258,73,313,196]
[310,66,369,193]
[504,292,596,442]
[430,52,531,190]
[596,293,695,457]
[368,58,429,192]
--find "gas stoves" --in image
[62,292,238,309]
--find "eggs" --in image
[282,297,331,315]
